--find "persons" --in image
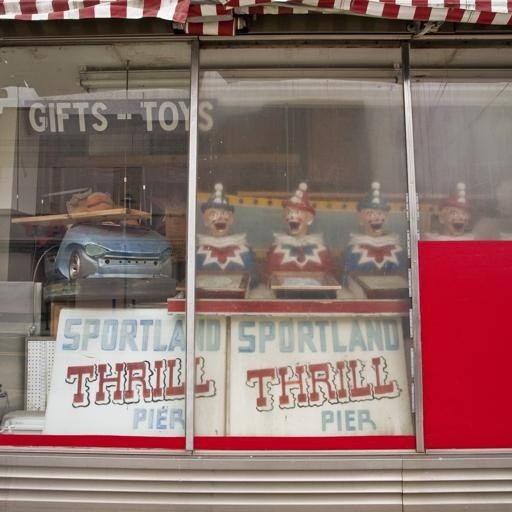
[260,182,332,284]
[340,181,407,286]
[420,184,476,242]
[197,182,259,289]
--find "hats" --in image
[280,182,316,213]
[200,183,235,211]
[440,182,474,211]
[355,182,391,211]
